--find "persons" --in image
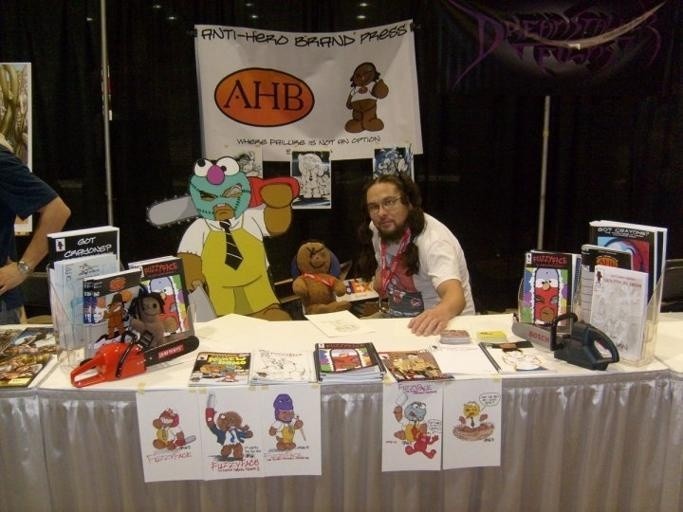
[0,145,73,325]
[356,172,477,337]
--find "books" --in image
[514,214,667,367]
[188,341,455,384]
[0,222,199,389]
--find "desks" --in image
[0,325,69,512]
[649,315,682,512]
[39,318,671,512]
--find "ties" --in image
[219,218,245,273]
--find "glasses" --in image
[366,195,401,214]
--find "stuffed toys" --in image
[289,238,353,316]
